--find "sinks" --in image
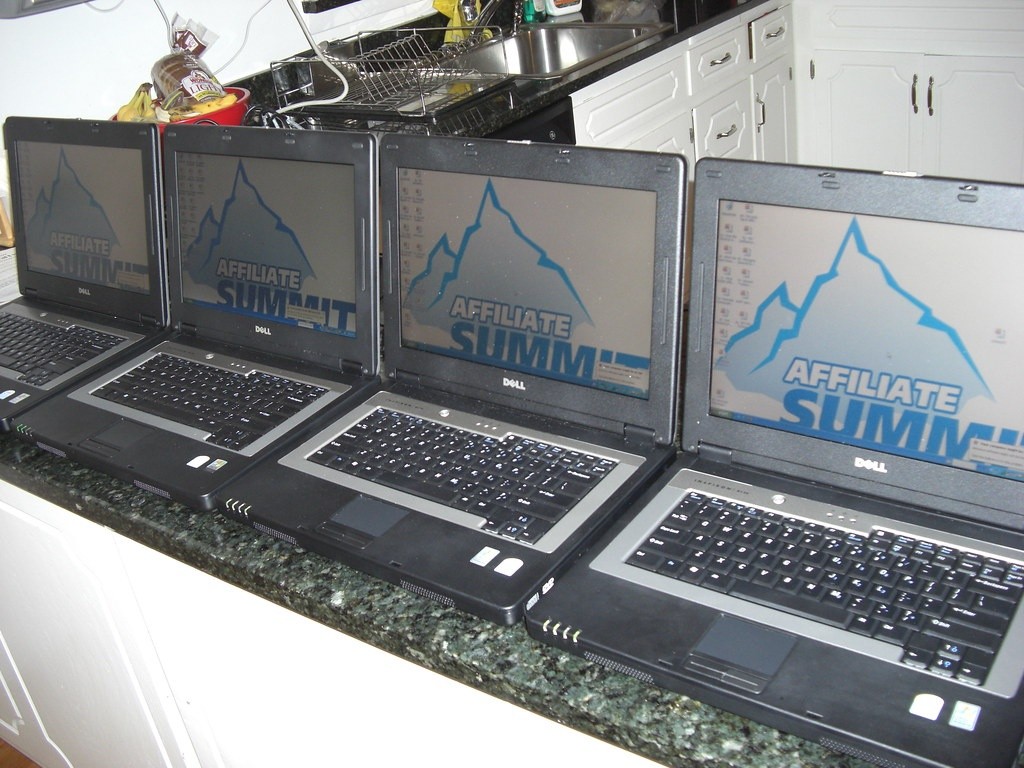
[391,23,675,80]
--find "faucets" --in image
[463,0,503,43]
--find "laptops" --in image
[0,116,1024,768]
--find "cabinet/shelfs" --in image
[568,4,1024,185]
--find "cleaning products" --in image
[523,0,582,23]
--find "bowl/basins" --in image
[110,87,250,134]
